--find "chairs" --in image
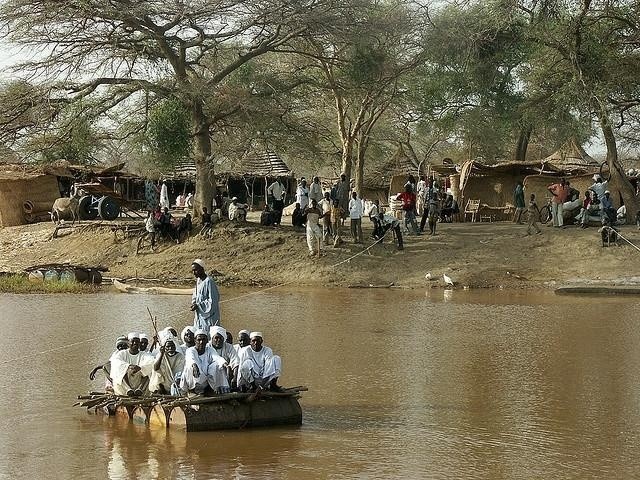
[464,197,481,222]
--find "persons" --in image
[511,184,525,225]
[89,325,283,399]
[189,258,221,342]
[547,173,628,230]
[523,193,541,237]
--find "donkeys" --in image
[47,187,81,226]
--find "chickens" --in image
[443,273,454,286]
[425,271,432,281]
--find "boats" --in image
[26,263,109,284]
[72,386,311,432]
[111,279,194,296]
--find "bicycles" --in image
[539,195,554,225]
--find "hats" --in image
[193,258,205,267]
[116,326,263,347]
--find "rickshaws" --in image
[76,181,144,222]
